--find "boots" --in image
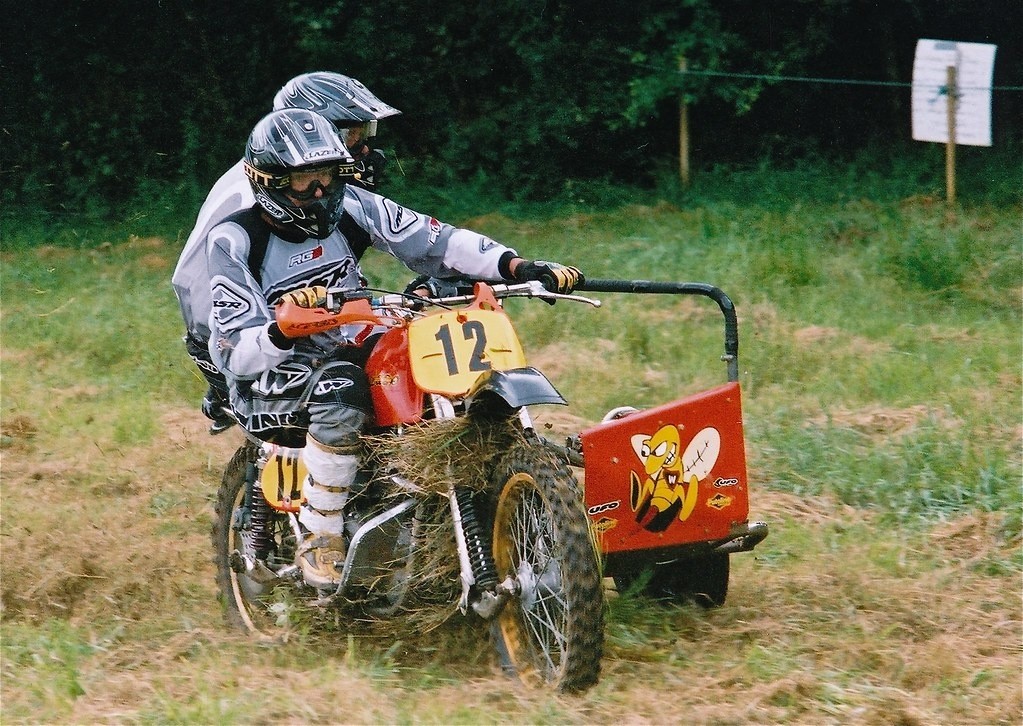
[296,432,363,589]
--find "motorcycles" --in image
[212,272,606,697]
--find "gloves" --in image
[515,261,585,307]
[277,285,328,308]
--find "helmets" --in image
[244,108,354,240]
[273,70,401,180]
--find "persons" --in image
[207,107,586,591]
[171,72,400,397]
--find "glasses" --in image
[334,120,378,155]
[286,165,342,199]
